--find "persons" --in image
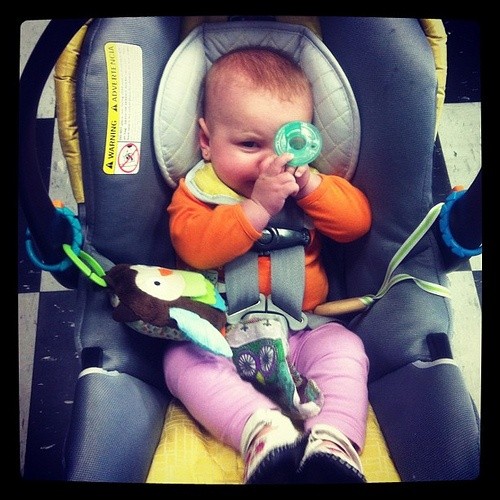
[162,47,372,484]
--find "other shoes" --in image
[296,425,365,482]
[240,409,303,484]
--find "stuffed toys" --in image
[102,263,234,359]
